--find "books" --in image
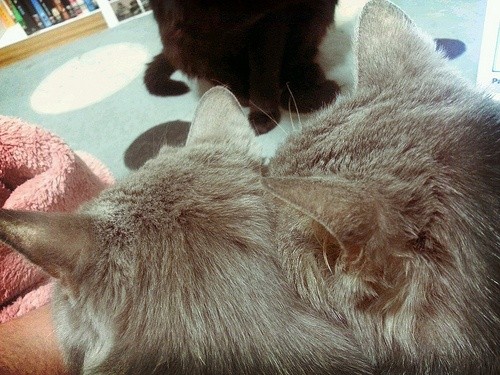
[7,0,98,36]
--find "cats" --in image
[0,86,374,375]
[257,1,500,375]
[142,0,342,135]
